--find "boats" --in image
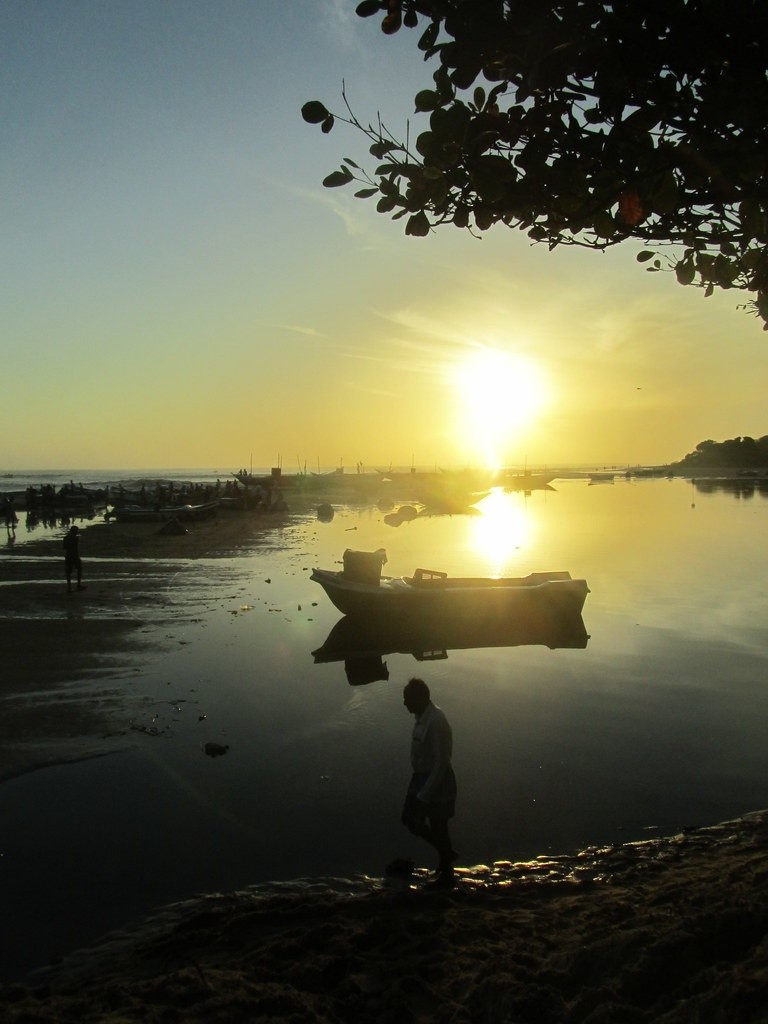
[309,455,393,481]
[412,491,493,507]
[587,473,615,480]
[310,614,592,664]
[230,452,307,487]
[496,469,559,484]
[374,455,454,481]
[118,502,221,520]
[505,485,556,496]
[309,566,592,613]
[418,508,484,518]
[633,470,664,476]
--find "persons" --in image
[62,526,87,592]
[397,678,457,886]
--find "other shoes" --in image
[435,849,458,873]
[419,874,459,894]
